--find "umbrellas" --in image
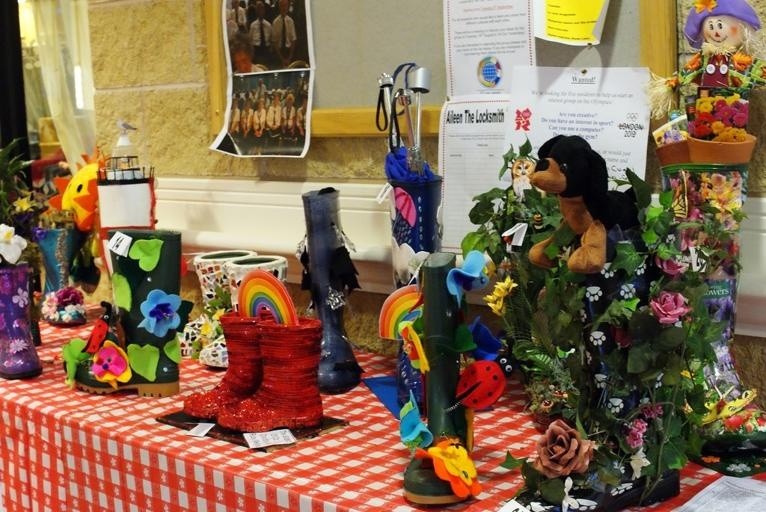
[399,62,442,188]
[376,71,408,184]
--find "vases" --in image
[655,132,757,166]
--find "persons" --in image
[223,1,309,155]
[646,1,766,144]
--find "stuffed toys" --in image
[44,146,108,232]
[525,135,634,275]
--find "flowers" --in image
[191,285,232,362]
[136,288,182,338]
[42,285,89,321]
[688,92,750,142]
[458,140,766,511]
[0,137,60,265]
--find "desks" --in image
[0,298,765,512]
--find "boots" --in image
[183,312,324,433]
[661,131,765,436]
[512,262,679,511]
[405,252,473,508]
[62,229,181,398]
[42,228,88,331]
[0,266,43,379]
[300,186,361,395]
[392,174,443,416]
[180,251,289,372]
